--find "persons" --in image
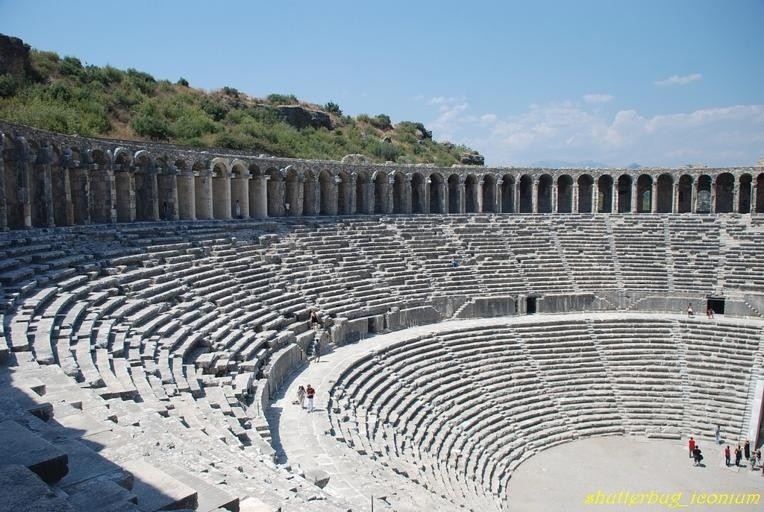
[707,307,715,319]
[306,384,315,413]
[714,425,721,444]
[235,200,241,218]
[687,303,692,318]
[110,205,118,224]
[724,440,762,471]
[297,385,306,409]
[313,338,321,363]
[310,311,320,331]
[162,202,169,221]
[284,201,290,217]
[693,445,702,466]
[688,437,695,458]
